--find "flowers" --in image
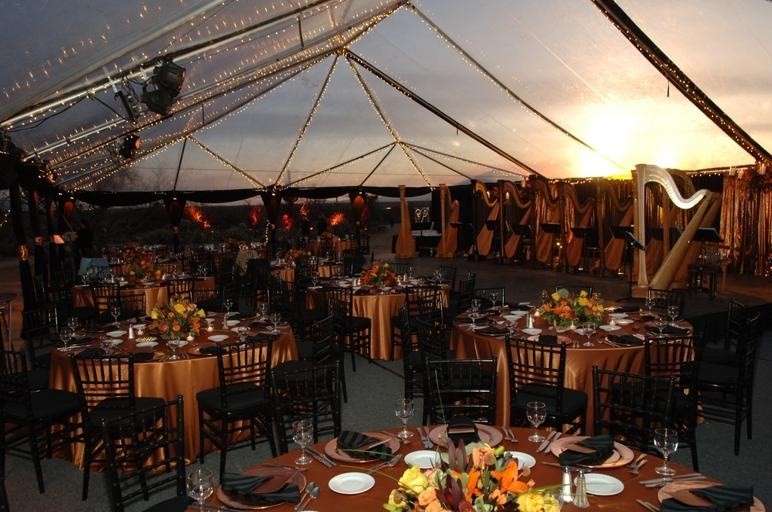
[383,442,564,512]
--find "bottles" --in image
[559,463,590,508]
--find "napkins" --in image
[220,471,302,505]
[335,430,393,461]
[445,414,480,449]
[559,434,613,467]
[659,484,756,511]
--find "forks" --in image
[368,454,402,474]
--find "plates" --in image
[325,432,400,463]
[103,318,252,348]
[605,333,646,345]
[429,423,504,449]
[328,472,376,495]
[527,335,572,346]
[550,436,635,469]
[216,462,307,510]
[502,302,634,335]
[573,472,625,496]
[472,325,514,336]
[503,450,536,471]
[657,480,766,512]
[404,450,442,469]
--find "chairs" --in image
[2,229,772,430]
[613,331,702,472]
[94,392,198,511]
[592,363,678,466]
[700,312,763,454]
[501,332,588,436]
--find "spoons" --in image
[501,426,520,443]
[294,482,320,512]
[626,453,648,474]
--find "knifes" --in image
[536,430,562,454]
[417,426,434,449]
[640,472,707,488]
[304,446,337,468]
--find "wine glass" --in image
[583,322,596,347]
[60,327,72,352]
[186,468,215,512]
[100,337,113,362]
[526,401,547,442]
[668,306,679,327]
[489,289,499,310]
[271,313,282,333]
[468,307,480,328]
[167,335,182,360]
[653,427,680,476]
[259,302,269,321]
[646,296,655,315]
[68,317,79,337]
[655,314,667,338]
[394,398,416,438]
[111,307,121,325]
[223,298,234,317]
[292,419,314,464]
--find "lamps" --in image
[140,62,185,116]
[119,133,142,159]
